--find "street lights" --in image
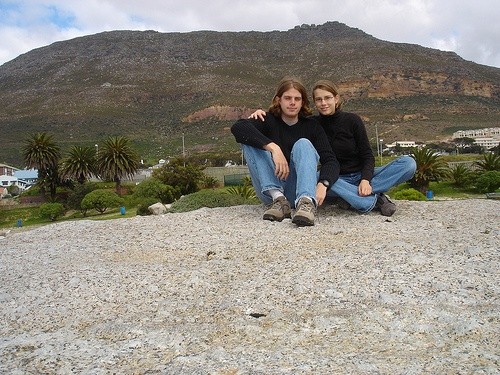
[374,125,379,157]
[379,139,382,167]
[95,144,99,164]
[182,132,185,168]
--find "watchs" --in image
[320,180,330,187]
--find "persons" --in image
[230,81,340,226]
[247,81,417,217]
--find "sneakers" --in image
[292,198,316,226]
[376,193,396,217]
[340,201,353,209]
[263,196,291,221]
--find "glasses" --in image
[315,96,335,103]
